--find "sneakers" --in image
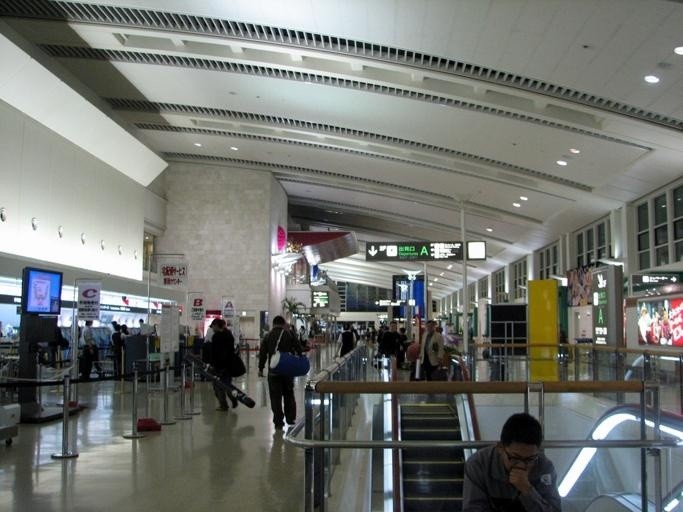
[275,421,284,430]
[215,405,228,411]
[286,416,295,425]
[231,398,238,409]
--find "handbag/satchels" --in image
[223,353,246,378]
[432,342,439,353]
[256,375,267,408]
[414,358,422,381]
[270,351,311,377]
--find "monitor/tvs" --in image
[21,265,63,315]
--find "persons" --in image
[299,325,308,347]
[69,317,146,379]
[331,320,450,403]
[209,317,238,412]
[199,318,212,382]
[289,324,302,355]
[221,320,234,349]
[637,308,651,345]
[258,313,302,432]
[462,410,563,511]
[655,308,672,346]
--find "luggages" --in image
[431,370,447,381]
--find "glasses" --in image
[503,447,540,465]
